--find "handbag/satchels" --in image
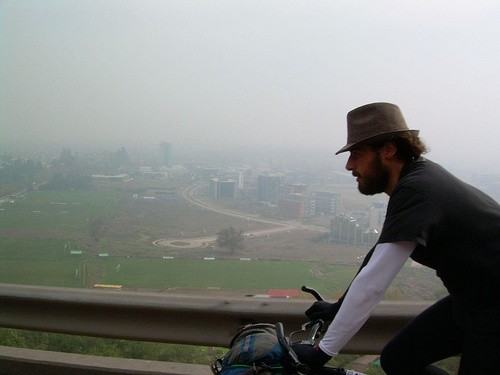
[212,324,297,375]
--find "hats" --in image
[335,102,421,156]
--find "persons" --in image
[291,102,500,375]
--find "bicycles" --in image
[207,286,348,375]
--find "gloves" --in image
[281,345,328,370]
[304,300,340,320]
